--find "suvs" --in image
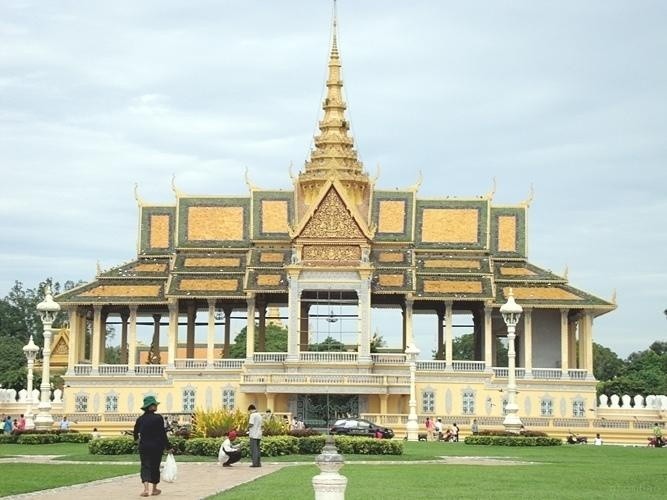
[258,412,305,432]
[327,419,396,440]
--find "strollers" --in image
[565,427,588,446]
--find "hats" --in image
[139,396,160,411]
[227,431,236,438]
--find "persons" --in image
[373,429,383,438]
[59,416,68,431]
[471,419,478,435]
[592,433,603,445]
[0,412,26,435]
[290,416,299,430]
[218,431,243,467]
[245,403,263,467]
[164,415,174,434]
[424,417,459,443]
[91,428,99,439]
[261,408,275,420]
[297,417,306,428]
[281,415,288,425]
[652,423,662,448]
[132,395,175,497]
[567,435,577,444]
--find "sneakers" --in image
[249,463,261,467]
[139,490,161,496]
[222,464,232,467]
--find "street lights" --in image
[35,286,61,430]
[497,285,524,433]
[21,335,40,431]
[402,340,422,442]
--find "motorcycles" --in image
[647,437,667,447]
